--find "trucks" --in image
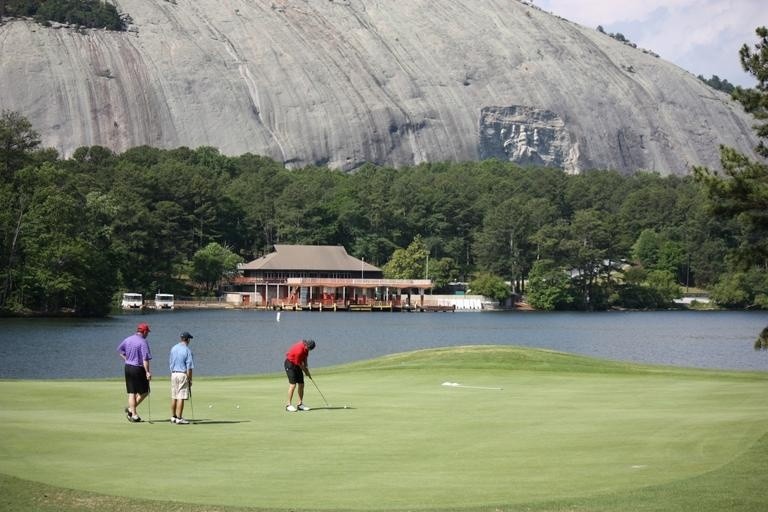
[121,293,144,312]
[154,293,175,311]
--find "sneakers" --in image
[176,418,190,424]
[286,405,298,412]
[171,416,183,423]
[131,416,146,423]
[125,407,132,423]
[297,404,310,411]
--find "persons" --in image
[116,323,153,423]
[169,331,195,425]
[284,338,316,413]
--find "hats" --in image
[302,339,316,351]
[180,331,193,339]
[137,323,150,333]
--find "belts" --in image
[172,371,186,374]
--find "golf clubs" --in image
[310,376,332,407]
[148,383,154,424]
[188,377,198,424]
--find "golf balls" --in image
[344,405,347,409]
[237,406,240,408]
[209,405,212,408]
